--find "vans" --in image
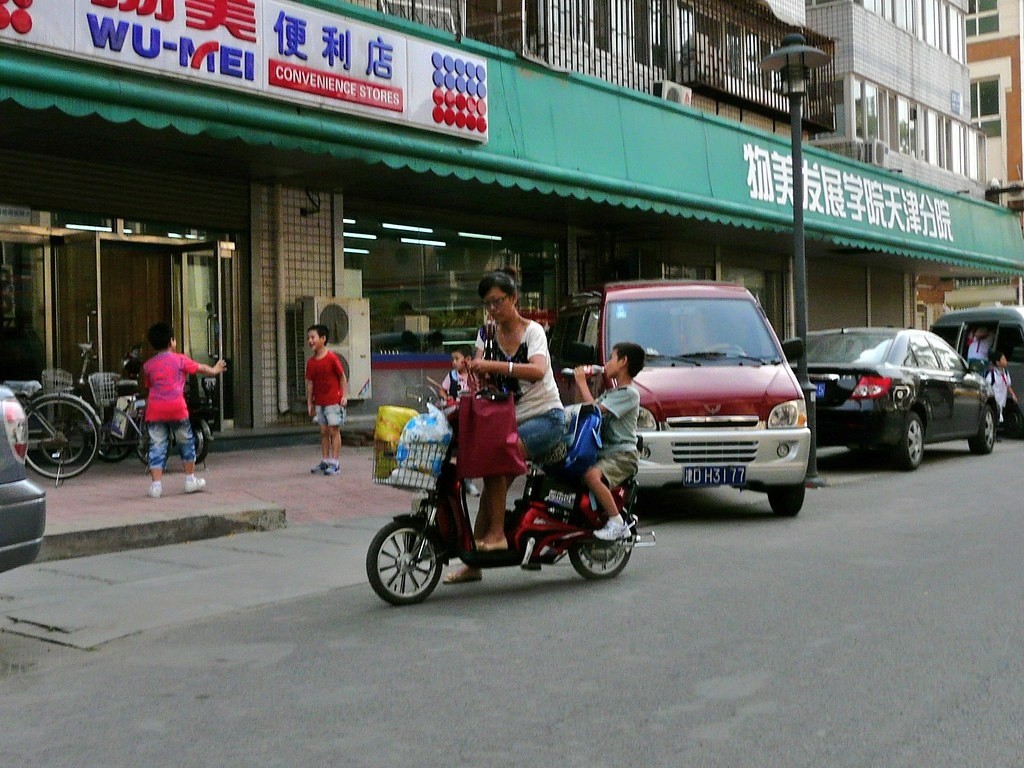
[545,280,812,518]
[930,306,1024,439]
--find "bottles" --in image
[561,364,604,383]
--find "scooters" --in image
[365,382,657,606]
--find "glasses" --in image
[479,293,508,308]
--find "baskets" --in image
[371,441,449,492]
[88,372,121,407]
[42,368,72,394]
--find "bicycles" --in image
[4,343,212,489]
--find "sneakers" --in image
[311,461,328,472]
[148,486,162,498]
[185,478,206,493]
[324,463,340,476]
[593,521,631,541]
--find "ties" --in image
[1002,373,1007,385]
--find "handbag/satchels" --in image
[456,369,529,480]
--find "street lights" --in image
[757,33,835,484]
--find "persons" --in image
[967,328,994,361]
[983,352,1018,422]
[574,343,645,542]
[305,325,347,475]
[143,322,227,499]
[444,268,567,582]
[439,345,479,498]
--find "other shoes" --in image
[443,569,482,583]
[476,540,509,549]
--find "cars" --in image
[787,325,1000,470]
[1,384,47,573]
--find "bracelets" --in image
[509,362,513,373]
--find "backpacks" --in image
[541,401,603,475]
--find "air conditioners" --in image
[650,79,693,108]
[293,294,373,404]
[861,137,891,169]
